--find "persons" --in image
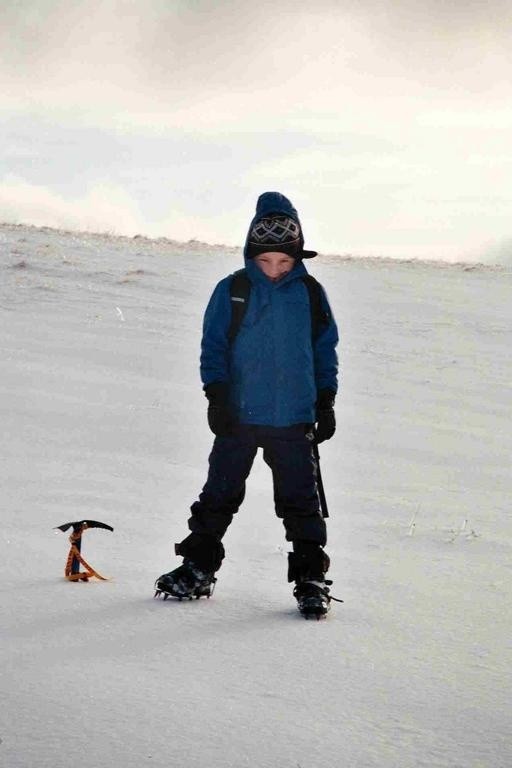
[156,192,339,614]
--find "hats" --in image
[246,212,317,260]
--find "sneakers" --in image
[293,574,344,621]
[154,561,215,601]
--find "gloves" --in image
[315,389,336,443]
[203,381,234,439]
